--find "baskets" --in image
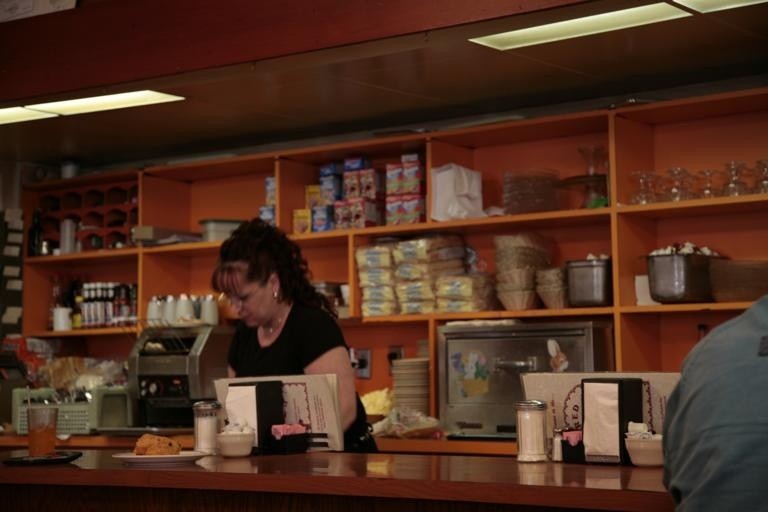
[497,244,568,310]
[16,403,91,435]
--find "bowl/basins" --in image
[391,357,431,419]
[625,438,667,467]
[627,467,668,493]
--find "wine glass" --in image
[629,158,767,204]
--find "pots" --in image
[644,254,728,304]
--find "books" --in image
[213,373,344,451]
[519,372,681,438]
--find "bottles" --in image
[60,219,76,256]
[514,399,563,463]
[518,463,563,487]
[72,281,137,330]
[193,400,221,457]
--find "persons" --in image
[662,295,767,508]
[210,218,378,453]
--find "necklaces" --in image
[262,314,283,336]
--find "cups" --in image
[216,433,255,458]
[143,294,220,327]
[27,408,58,456]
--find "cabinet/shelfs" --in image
[0,87,768,454]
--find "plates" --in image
[5,452,83,465]
[111,450,217,465]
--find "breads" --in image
[134,432,183,455]
